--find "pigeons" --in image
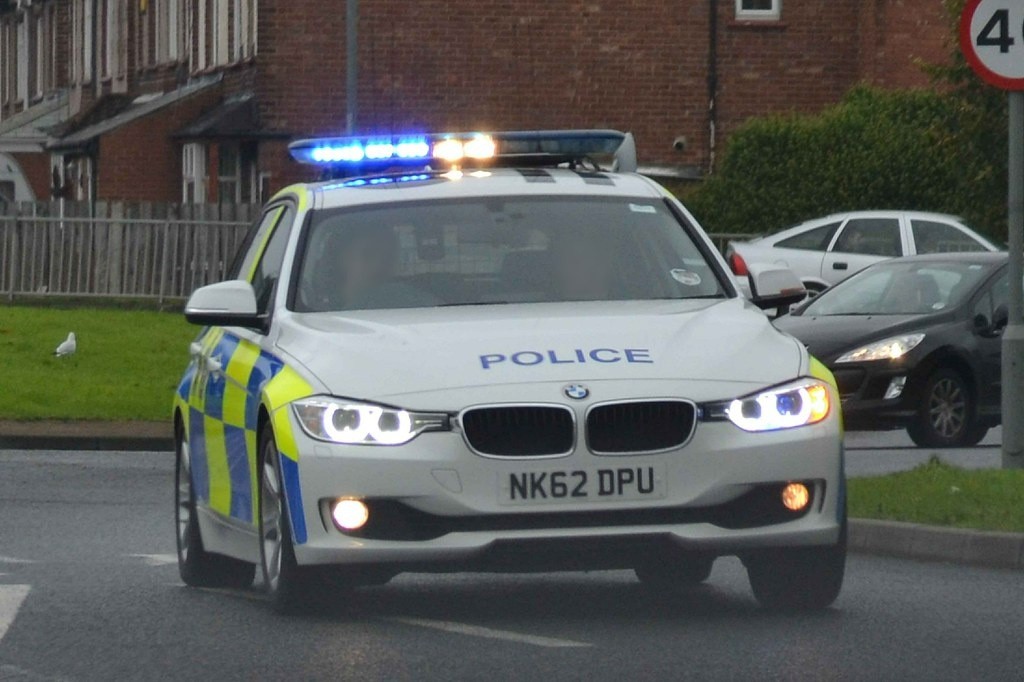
[51,332,76,357]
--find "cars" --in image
[722,210,1000,319]
[767,252,1009,449]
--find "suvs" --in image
[170,128,845,616]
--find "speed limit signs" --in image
[960,1,1024,90]
